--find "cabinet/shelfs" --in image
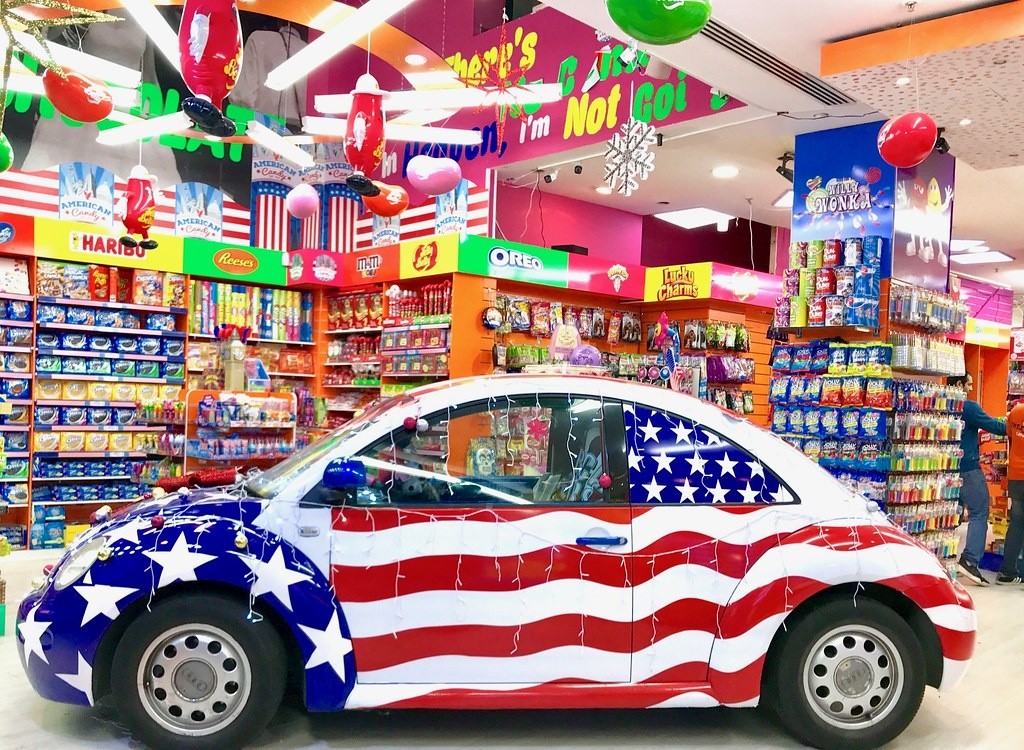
[497,287,775,478]
[0,249,318,551]
[325,272,497,482]
[764,284,1024,582]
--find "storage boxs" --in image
[327,289,384,332]
[383,326,450,395]
[0,257,315,550]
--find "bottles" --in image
[223,335,245,392]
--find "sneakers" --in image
[995,572,1024,585]
[957,554,991,587]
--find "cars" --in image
[9,368,980,750]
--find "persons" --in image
[946,370,1007,587]
[996,404,1024,584]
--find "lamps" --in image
[0,25,142,89]
[776,151,794,183]
[574,163,583,174]
[120,0,181,73]
[303,116,484,145]
[934,127,950,153]
[97,110,192,147]
[244,120,314,169]
[543,168,559,183]
[314,82,562,113]
[263,0,414,92]
[617,46,638,68]
